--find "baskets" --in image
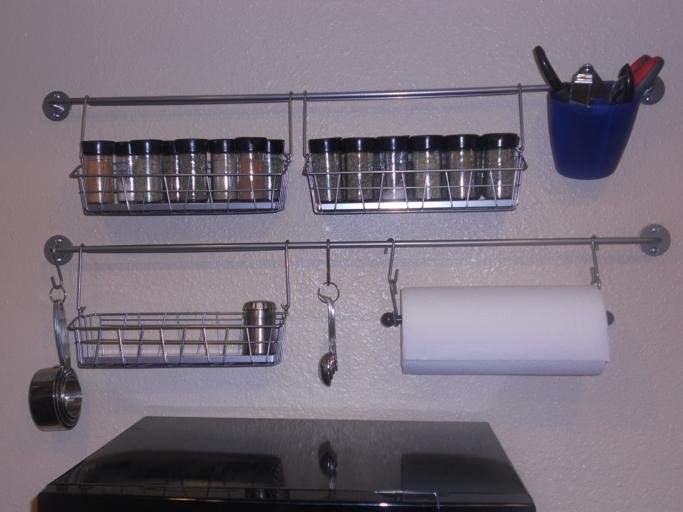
[307,157,526,209]
[77,315,283,365]
[77,166,285,216]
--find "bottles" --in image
[243,300,276,356]
[80,136,285,204]
[308,132,519,202]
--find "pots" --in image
[29,299,82,432]
